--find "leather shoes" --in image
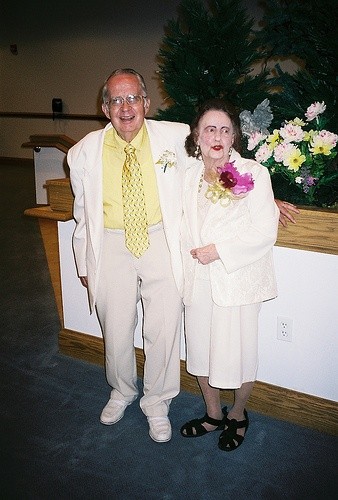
[147,415,172,443]
[99,399,132,425]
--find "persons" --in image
[177,99,281,452]
[65,69,299,443]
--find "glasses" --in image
[105,95,147,106]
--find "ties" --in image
[122,144,150,259]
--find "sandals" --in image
[218,408,248,451]
[180,406,228,437]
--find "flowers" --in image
[156,150,176,173]
[204,159,255,206]
[241,97,338,207]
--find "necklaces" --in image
[197,172,211,207]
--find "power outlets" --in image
[277,317,293,341]
